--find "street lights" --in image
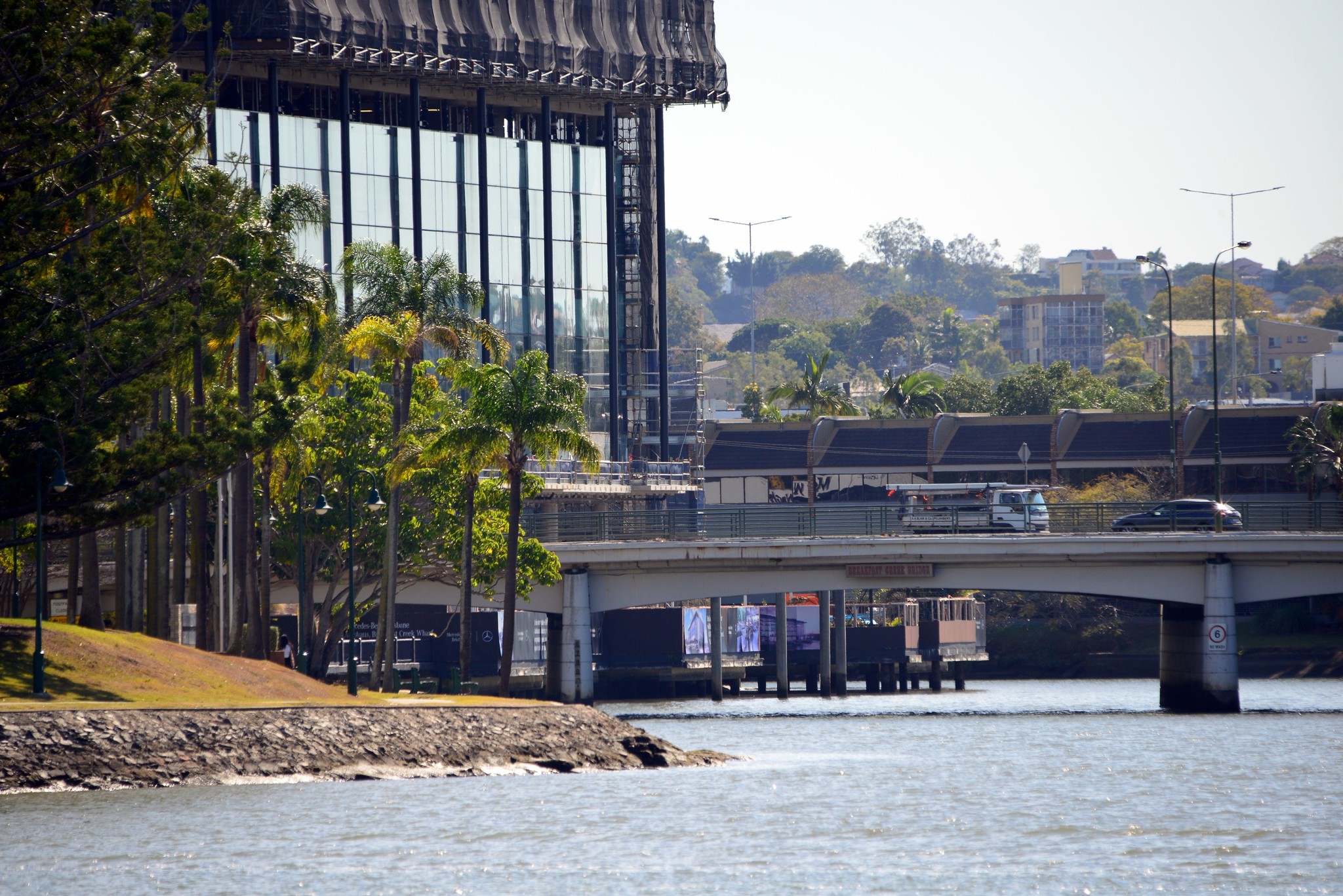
[709,216,792,387]
[1178,186,1287,404]
[348,469,386,700]
[1211,240,1253,534]
[1135,255,1178,532]
[31,447,74,696]
[296,475,333,678]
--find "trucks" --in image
[898,483,1050,534]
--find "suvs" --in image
[1109,498,1244,533]
